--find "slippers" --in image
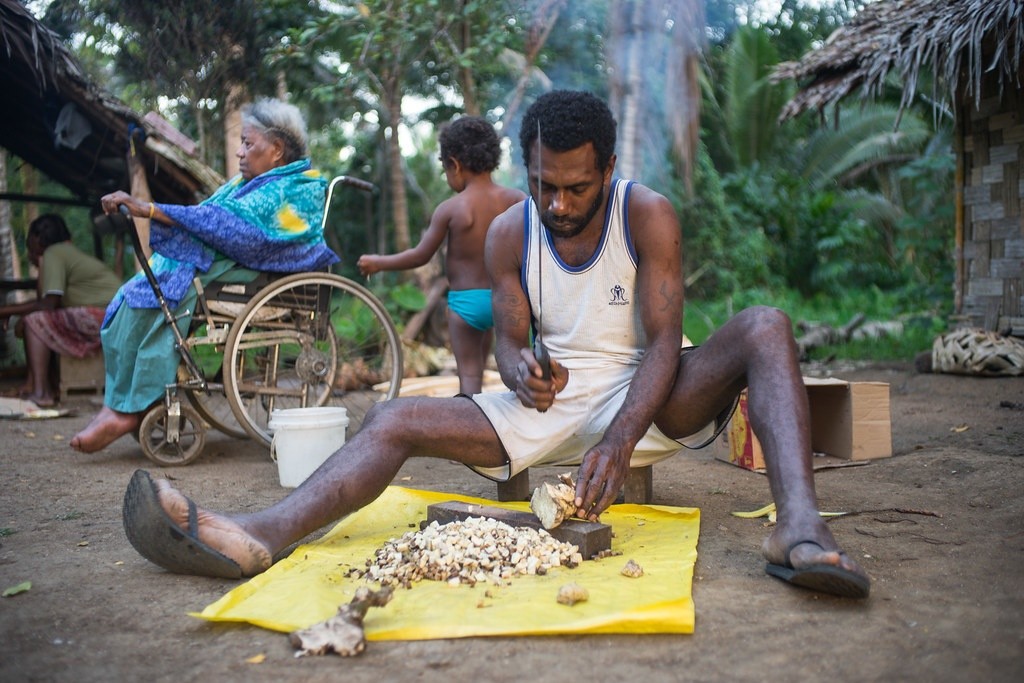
[763,538,872,600]
[120,467,244,583]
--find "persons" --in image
[0,214,123,407]
[122,90,873,602]
[71,97,327,454]
[356,117,528,393]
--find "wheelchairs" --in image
[105,174,405,466]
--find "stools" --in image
[497,464,653,505]
[61,345,106,404]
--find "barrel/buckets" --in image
[267,407,350,487]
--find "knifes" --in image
[534,120,552,410]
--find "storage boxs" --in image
[714,377,893,474]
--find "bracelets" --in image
[150,202,154,217]
